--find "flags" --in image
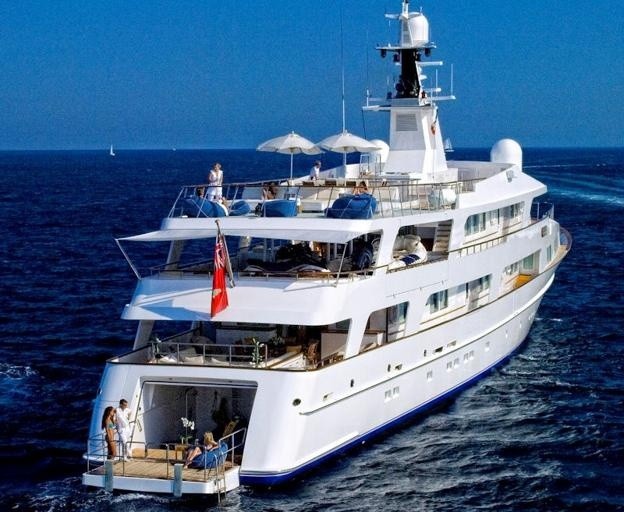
[208,231,228,319]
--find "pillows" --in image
[159,347,229,367]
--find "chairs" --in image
[180,181,401,220]
[187,440,229,470]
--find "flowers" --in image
[179,417,195,441]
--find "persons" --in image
[190,328,214,354]
[307,160,321,180]
[351,181,370,196]
[197,189,204,198]
[100,406,116,460]
[206,163,222,200]
[182,430,221,469]
[259,181,274,200]
[112,399,132,460]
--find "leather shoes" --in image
[120,450,131,460]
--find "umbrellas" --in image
[254,128,322,180]
[313,129,380,176]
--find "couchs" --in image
[148,347,306,370]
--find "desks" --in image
[359,169,422,202]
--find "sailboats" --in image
[109,143,115,157]
[444,137,454,154]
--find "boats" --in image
[74,2,577,497]
